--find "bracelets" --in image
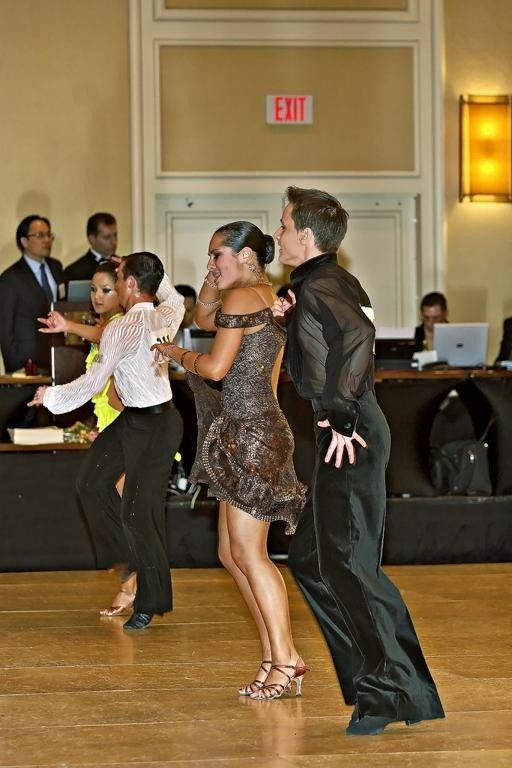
[181,350,191,368]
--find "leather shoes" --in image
[345,705,425,735]
[120,562,138,585]
[122,608,165,631]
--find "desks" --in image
[0,357,511,567]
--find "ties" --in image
[38,264,53,304]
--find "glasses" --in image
[27,231,57,241]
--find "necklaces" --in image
[247,281,272,288]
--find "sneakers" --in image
[99,589,136,617]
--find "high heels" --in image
[249,656,308,700]
[238,660,292,696]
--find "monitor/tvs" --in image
[374,339,422,359]
[433,322,489,368]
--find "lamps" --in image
[457,91,512,200]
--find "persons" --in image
[56,211,121,276]
[149,222,306,702]
[24,250,186,632]
[410,292,449,352]
[162,284,200,374]
[271,283,298,384]
[268,183,448,741]
[35,260,139,619]
[1,214,65,375]
